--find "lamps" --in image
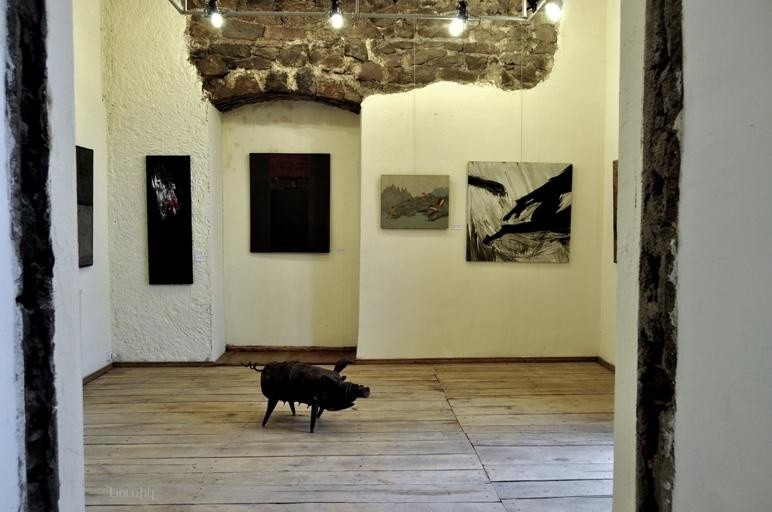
[169,1,562,36]
